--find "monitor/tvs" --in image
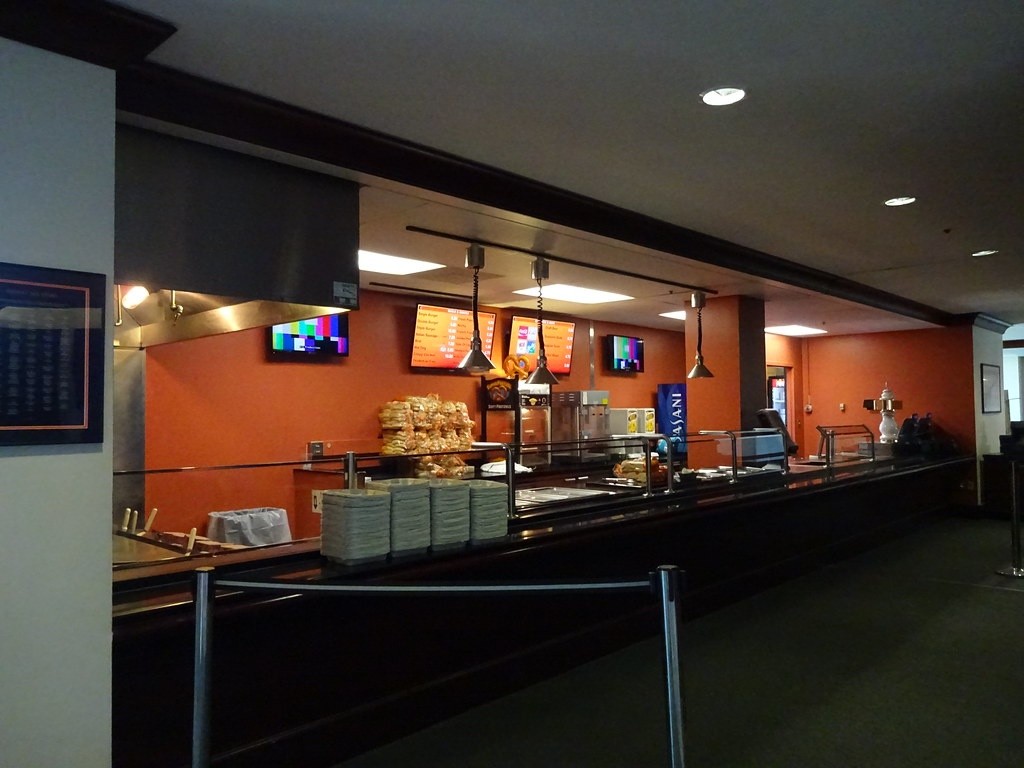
[269,313,350,357]
[607,334,644,373]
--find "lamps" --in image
[524,260,560,385]
[455,244,497,372]
[686,289,714,378]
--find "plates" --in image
[320,478,508,561]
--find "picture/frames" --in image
[980,363,1002,413]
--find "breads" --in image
[502,354,531,381]
[613,457,668,485]
[378,396,474,477]
[490,386,508,400]
